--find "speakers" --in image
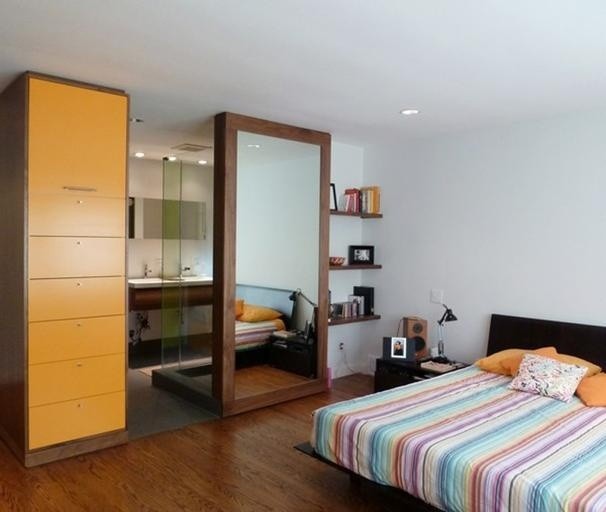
[404,318,427,361]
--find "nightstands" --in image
[263,335,316,377]
[371,358,474,394]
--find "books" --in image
[334,285,376,318]
[273,329,296,338]
[421,361,455,373]
[335,183,380,213]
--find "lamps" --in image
[289,288,318,345]
[434,304,457,362]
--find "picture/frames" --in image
[390,337,407,359]
[303,320,311,341]
[349,245,374,265]
[330,183,338,211]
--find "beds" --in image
[310,314,605,511]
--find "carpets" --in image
[294,441,362,479]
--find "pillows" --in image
[235,299,243,318]
[473,346,557,377]
[575,372,604,406]
[557,353,602,378]
[506,352,588,403]
[238,305,284,322]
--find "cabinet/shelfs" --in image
[327,209,383,327]
[0,70,129,468]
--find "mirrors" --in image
[211,112,331,418]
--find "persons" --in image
[392,340,404,356]
